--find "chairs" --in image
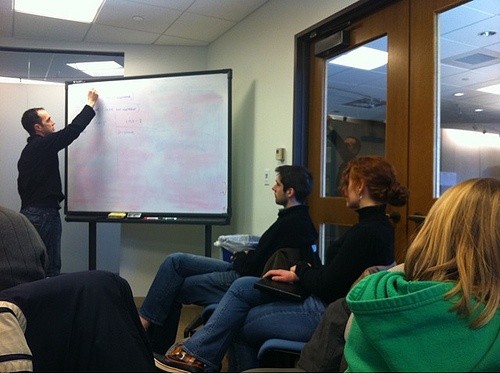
[183,263,397,373]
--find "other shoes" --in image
[154,345,217,373]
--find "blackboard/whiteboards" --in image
[66,68,231,219]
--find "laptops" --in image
[253,277,307,303]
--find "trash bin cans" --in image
[219,235,260,264]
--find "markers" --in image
[144,216,158,219]
[161,217,177,220]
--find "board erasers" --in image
[127,213,142,219]
[107,212,126,219]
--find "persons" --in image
[139,165,319,355]
[0,206,47,289]
[154,156,409,373]
[16,86,99,280]
[327,125,362,196]
[342,178,500,373]
